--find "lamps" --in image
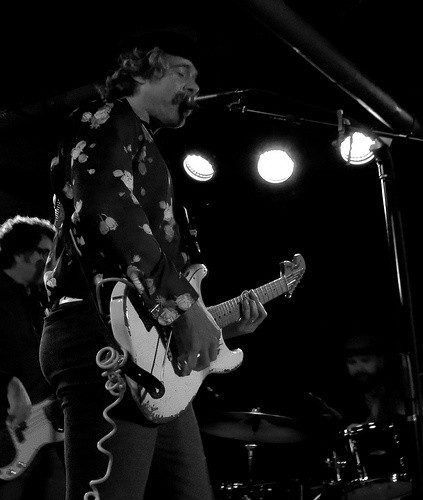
[329,110,378,165]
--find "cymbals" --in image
[196,411,303,443]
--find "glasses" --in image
[34,247,50,259]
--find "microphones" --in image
[181,88,246,109]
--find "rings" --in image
[250,320,255,323]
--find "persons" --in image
[39,37,268,499]
[0,215,67,500]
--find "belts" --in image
[57,295,83,305]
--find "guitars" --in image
[0,392,64,481]
[108,252,306,427]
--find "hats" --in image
[120,28,192,58]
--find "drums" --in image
[331,413,414,493]
